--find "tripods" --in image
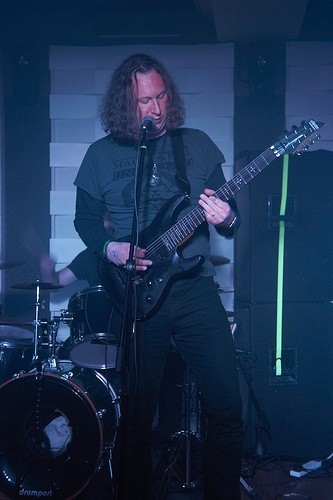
[141,366,260,500]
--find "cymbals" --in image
[210,255,230,266]
[0,261,25,269]
[10,283,64,290]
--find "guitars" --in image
[96,118,324,320]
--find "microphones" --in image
[139,116,156,133]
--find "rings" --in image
[133,256,135,261]
[212,212,216,217]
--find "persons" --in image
[72,53,250,499]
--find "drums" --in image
[67,284,119,370]
[0,360,122,500]
[0,323,34,385]
